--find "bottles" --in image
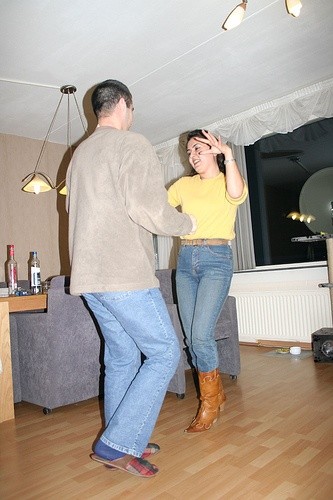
[5,244,18,295]
[29,250,41,295]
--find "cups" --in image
[43,281,50,293]
[290,347,302,355]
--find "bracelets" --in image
[222,156,237,164]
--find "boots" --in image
[185,369,221,432]
[216,368,226,413]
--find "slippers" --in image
[104,443,160,470]
[91,452,159,477]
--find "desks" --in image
[0,294,47,422]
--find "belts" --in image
[180,239,231,246]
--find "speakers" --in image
[311,328,333,363]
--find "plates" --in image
[276,349,290,354]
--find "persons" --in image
[166,128,249,431]
[63,77,197,477]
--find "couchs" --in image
[157,269,240,400]
[8,274,105,414]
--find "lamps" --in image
[219,0,249,32]
[21,85,90,196]
[285,0,303,17]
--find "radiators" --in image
[235,291,332,340]
